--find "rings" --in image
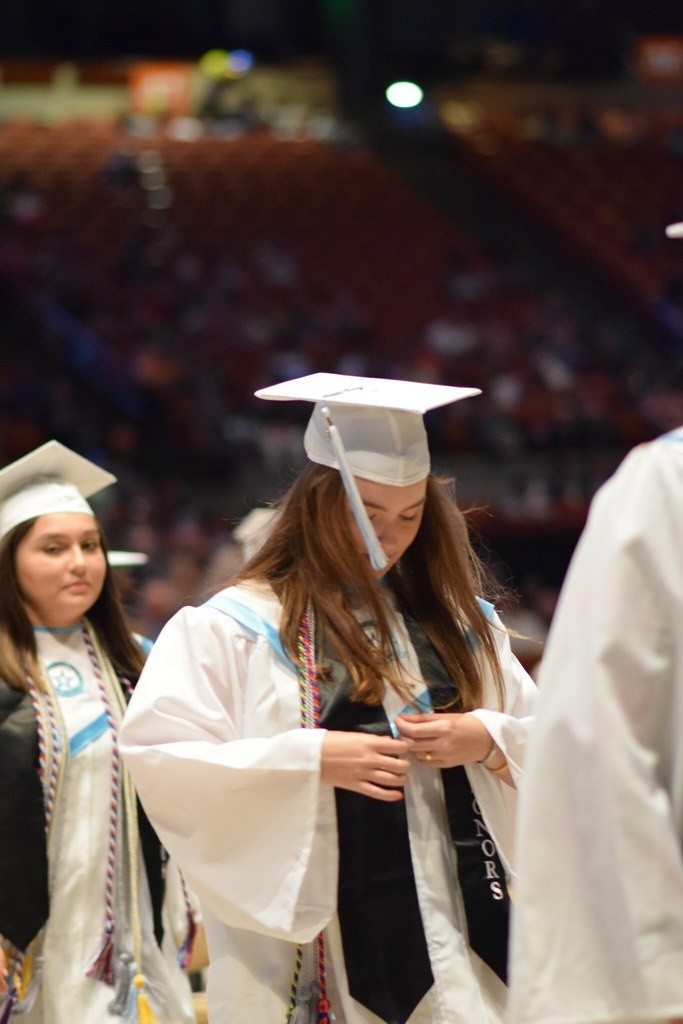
[425,752,433,761]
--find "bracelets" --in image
[484,762,508,773]
[476,741,499,766]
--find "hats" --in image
[233,508,283,561]
[254,372,482,571]
[0,440,118,539]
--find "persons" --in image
[117,374,539,1024]
[507,223,683,1024]
[0,438,204,1024]
